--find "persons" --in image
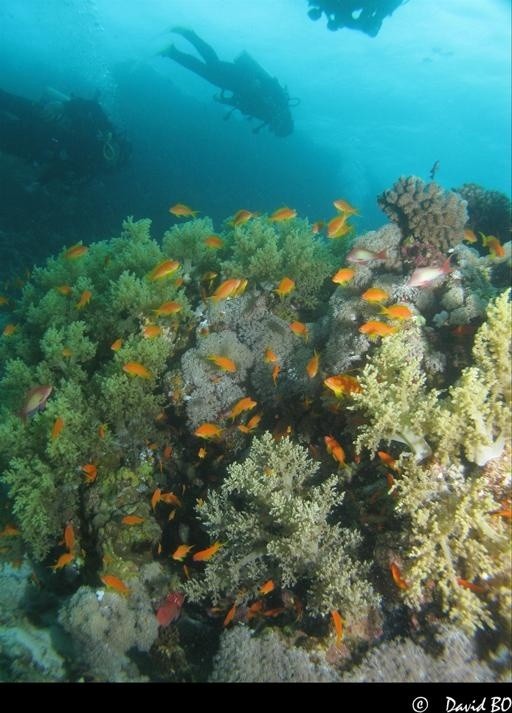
[159,25,300,137]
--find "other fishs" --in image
[0,197,511,646]
